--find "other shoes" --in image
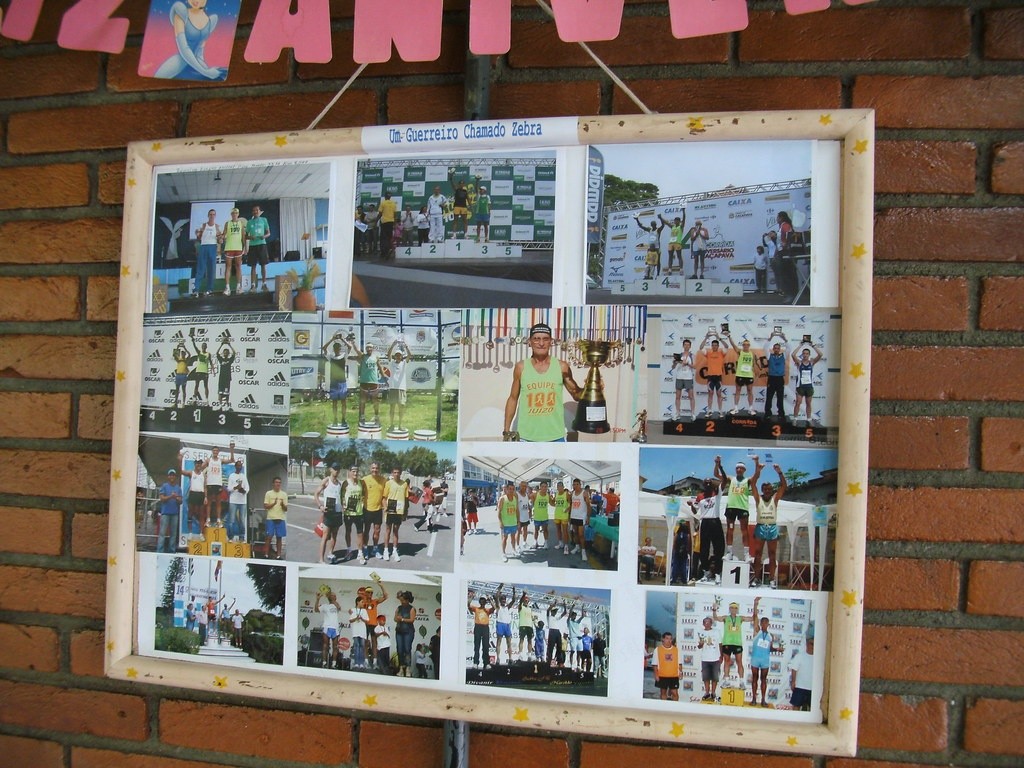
[322,661,411,677]
[203,291,214,296]
[262,284,269,293]
[236,286,245,295]
[472,656,607,678]
[249,285,257,294]
[191,292,199,298]
[702,681,769,707]
[667,268,704,279]
[672,407,815,427]
[223,288,232,296]
[687,551,776,589]
[188,520,281,560]
[468,529,587,562]
[318,511,448,565]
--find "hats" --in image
[480,186,487,191]
[168,469,176,475]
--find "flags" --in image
[188,557,195,576]
[214,560,222,582]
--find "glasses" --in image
[530,339,550,344]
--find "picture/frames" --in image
[103,109,875,755]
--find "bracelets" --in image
[319,505,324,509]
[713,608,716,611]
[503,431,512,436]
[720,338,722,341]
[685,363,688,365]
[812,343,816,348]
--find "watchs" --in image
[401,618,404,622]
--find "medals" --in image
[732,627,735,631]
[367,605,372,610]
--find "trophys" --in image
[777,640,786,655]
[697,632,707,645]
[715,594,723,611]
[568,336,623,435]
[632,409,647,444]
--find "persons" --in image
[160,171,821,710]
